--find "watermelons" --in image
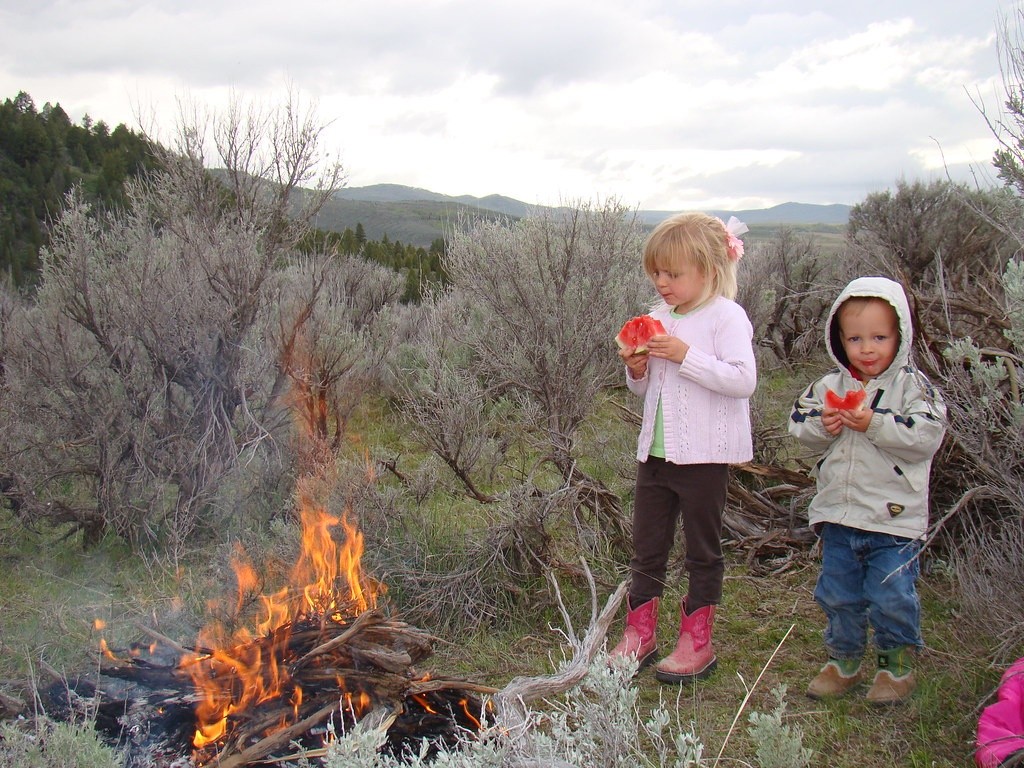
[824,388,868,417]
[614,314,670,353]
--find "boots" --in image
[656,595,717,682]
[607,593,659,677]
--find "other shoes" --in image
[865,667,916,705]
[805,655,869,700]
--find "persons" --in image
[606,211,757,684]
[788,276,947,704]
